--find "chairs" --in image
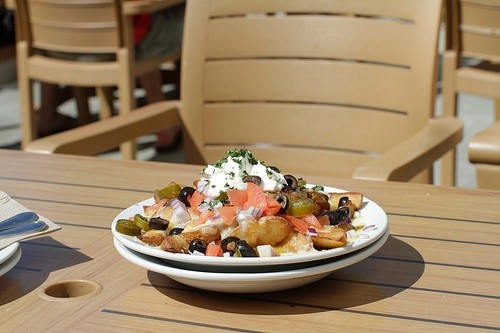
[440,0,500,185]
[15,0,181,159]
[24,0,464,183]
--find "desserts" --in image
[115,146,364,257]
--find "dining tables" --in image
[0,148,500,333]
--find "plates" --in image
[0,243,22,276]
[111,183,388,294]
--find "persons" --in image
[127,6,185,154]
